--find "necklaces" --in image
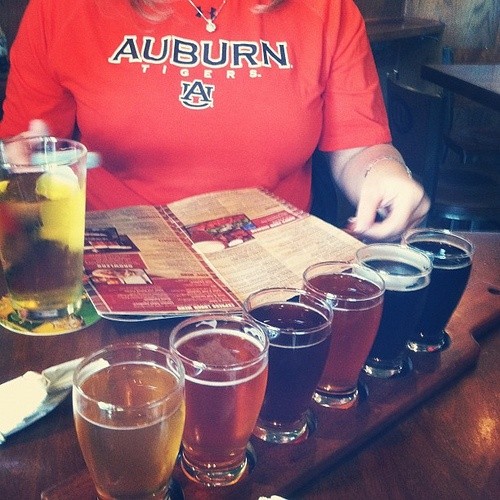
[189,0,225,33]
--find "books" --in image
[83,185,367,321]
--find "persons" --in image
[0,0,431,240]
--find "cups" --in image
[353,242,433,379]
[241,287,335,445]
[299,260,385,411]
[0,136,88,322]
[400,226,476,354]
[166,312,270,487]
[72,341,187,500]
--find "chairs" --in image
[311,66,499,228]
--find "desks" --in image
[409,63,500,164]
[367,12,449,44]
[0,231,500,500]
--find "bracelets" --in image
[365,156,412,177]
